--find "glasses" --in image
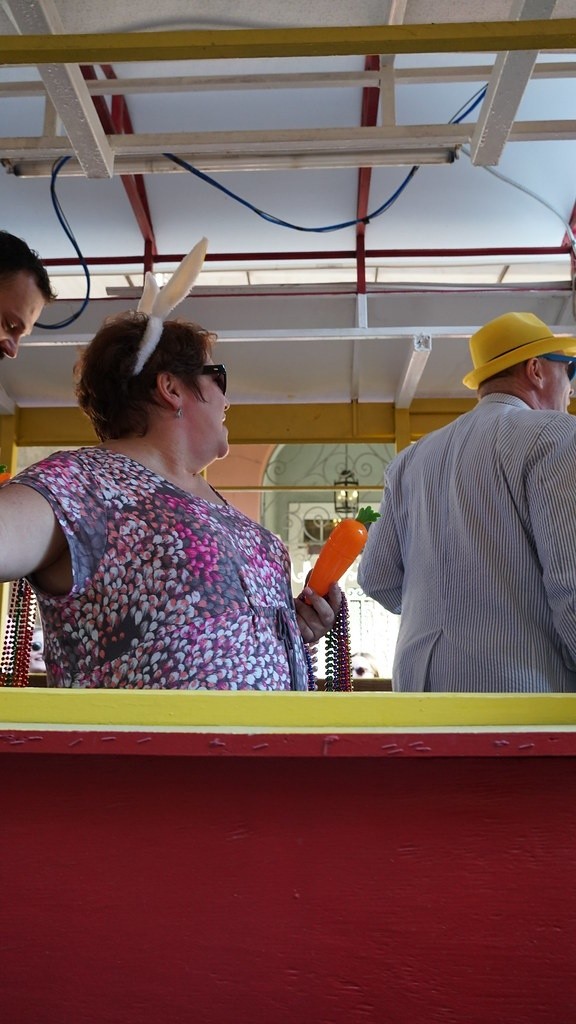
[164,363,227,396]
[536,353,576,381]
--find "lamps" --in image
[333,445,359,514]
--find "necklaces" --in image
[304,592,354,692]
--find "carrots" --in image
[304,505,381,605]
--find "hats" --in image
[462,311,576,391]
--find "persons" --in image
[0,236,342,690]
[357,312,576,693]
[0,230,58,361]
